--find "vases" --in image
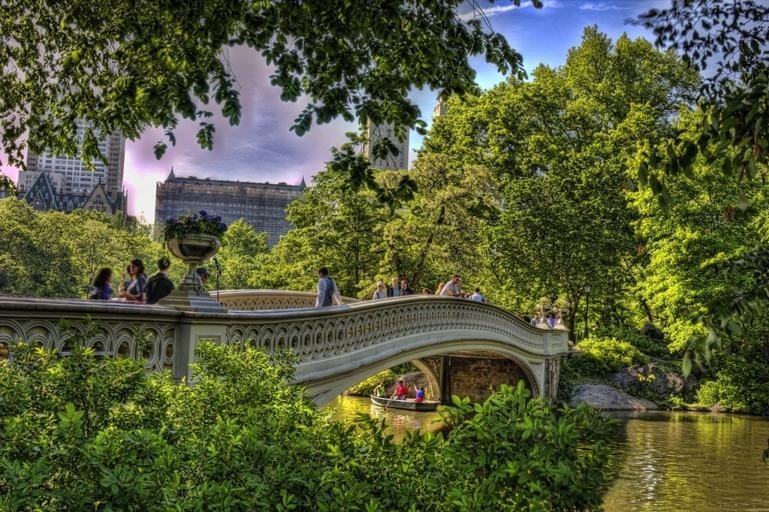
[180,233,212,256]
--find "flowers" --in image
[163,210,228,249]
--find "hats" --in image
[197,268,210,277]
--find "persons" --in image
[89,267,115,300]
[144,256,175,304]
[197,267,210,285]
[118,258,149,302]
[374,381,386,398]
[517,309,561,328]
[118,264,135,303]
[413,384,425,402]
[372,274,486,304]
[394,380,406,400]
[315,266,342,308]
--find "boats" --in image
[369,392,438,412]
